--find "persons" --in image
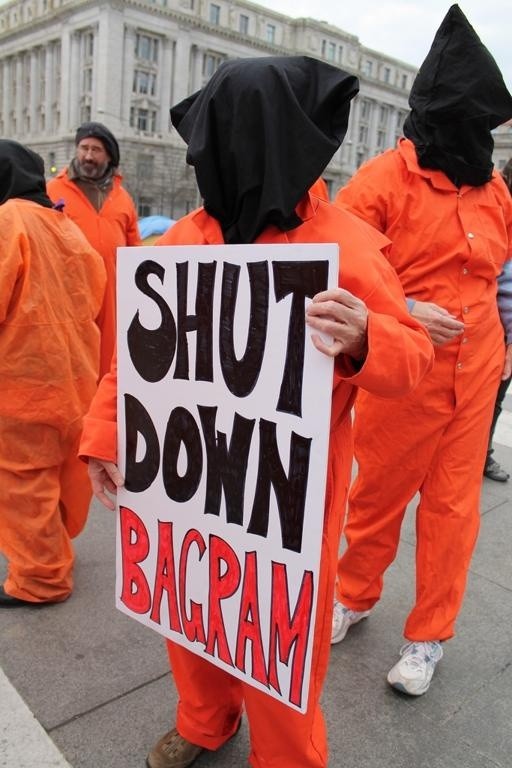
[46,122,143,378]
[78,56,433,768]
[0,140,106,605]
[332,3,512,697]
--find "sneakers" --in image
[387,640,442,694]
[330,602,373,644]
[146,730,203,767]
[487,460,507,483]
[0,587,63,603]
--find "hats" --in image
[75,121,119,168]
